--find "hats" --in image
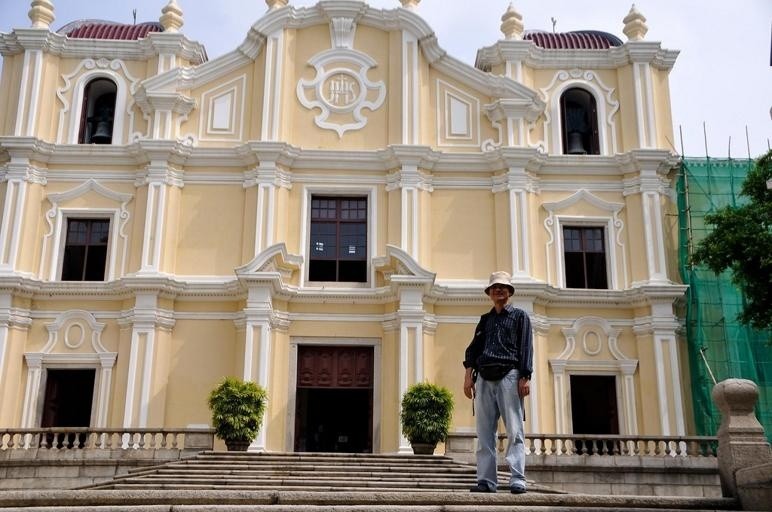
[484,272,514,297]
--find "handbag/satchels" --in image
[475,362,511,381]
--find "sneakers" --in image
[471,483,489,492]
[511,484,525,492]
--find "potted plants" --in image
[399,378,455,455]
[206,376,270,452]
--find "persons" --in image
[463,270,533,493]
